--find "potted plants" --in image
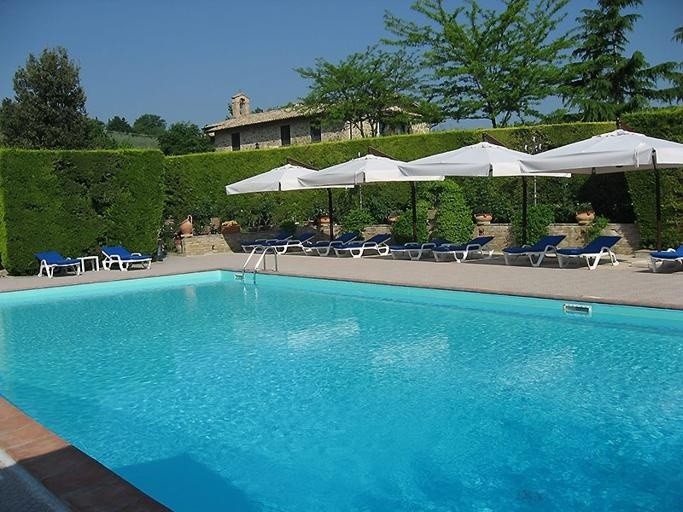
[574,202,596,226]
[472,206,492,226]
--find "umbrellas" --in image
[396,127,570,245]
[225,154,355,242]
[296,141,445,243]
[519,116,683,252]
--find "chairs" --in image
[390,236,497,263]
[501,235,622,271]
[32,246,152,280]
[241,233,315,255]
[302,234,392,258]
[649,244,682,275]
